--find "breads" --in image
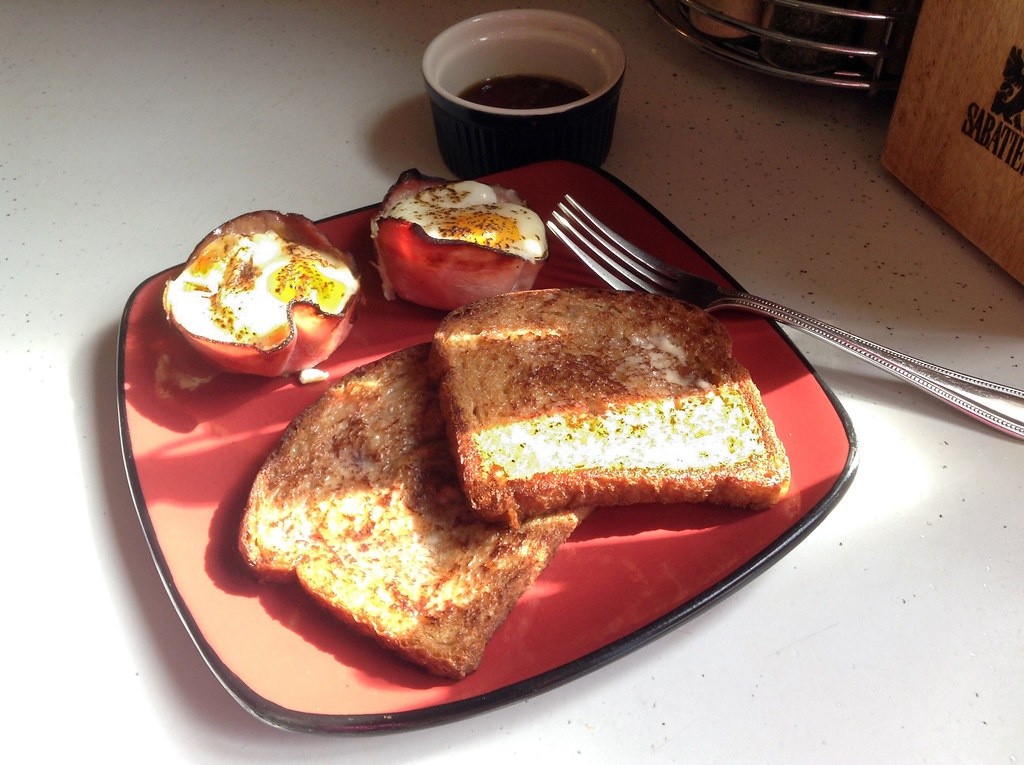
[431,289,790,530]
[238,341,595,680]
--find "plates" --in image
[117,158,859,737]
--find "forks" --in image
[546,195,1024,440]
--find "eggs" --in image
[166,230,360,350]
[383,178,547,263]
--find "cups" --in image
[421,9,626,179]
